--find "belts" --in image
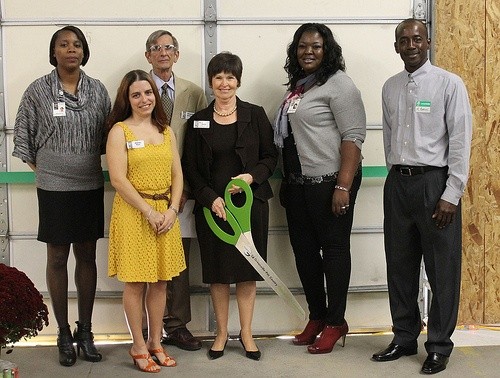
[391,166,439,176]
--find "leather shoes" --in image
[372,341,418,361]
[160,327,202,351]
[421,353,449,373]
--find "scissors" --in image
[203,178,307,322]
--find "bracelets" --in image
[334,184,349,192]
[146,207,154,221]
[169,204,179,215]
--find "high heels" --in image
[73,321,102,362]
[57,328,77,366]
[308,319,349,354]
[292,310,328,346]
[209,334,229,360]
[147,348,176,367]
[128,349,160,373]
[239,329,261,361]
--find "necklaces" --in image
[213,103,237,117]
[62,84,76,91]
[11,25,112,367]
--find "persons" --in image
[371,17,474,375]
[271,22,366,355]
[180,51,279,362]
[102,70,187,373]
[142,30,205,352]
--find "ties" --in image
[160,85,172,126]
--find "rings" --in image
[168,226,171,230]
[341,206,345,209]
[345,205,349,208]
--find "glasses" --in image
[150,45,176,52]
